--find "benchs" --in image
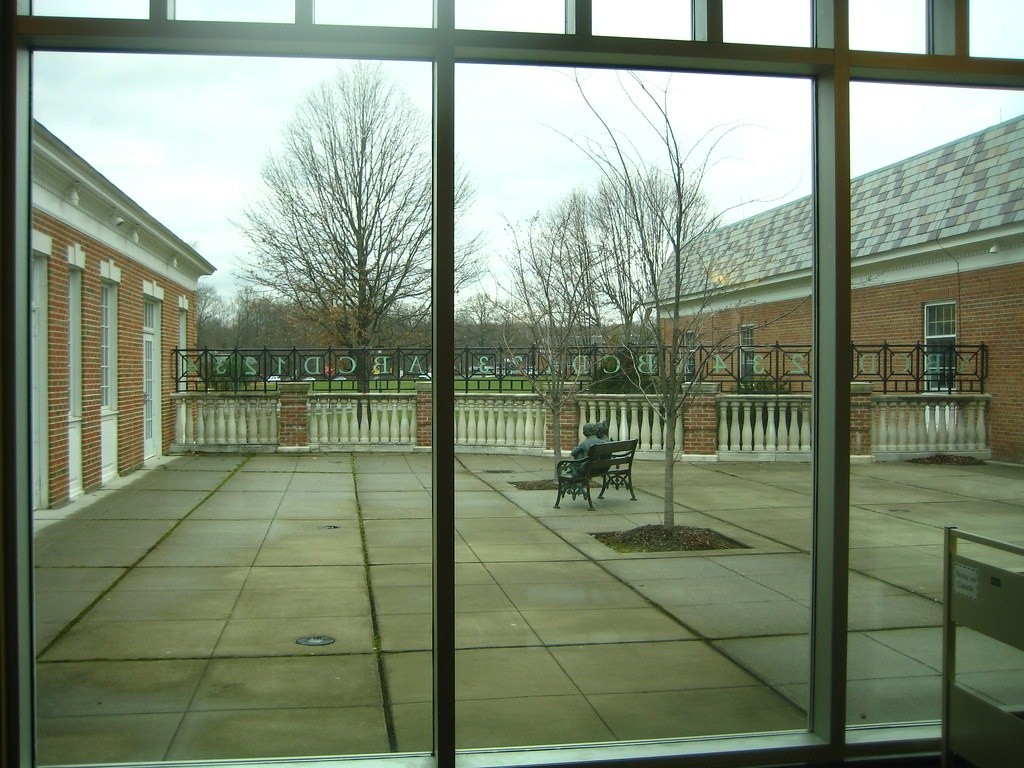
[554,438,639,511]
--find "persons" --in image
[566,420,612,476]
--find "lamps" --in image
[131,227,139,245]
[170,254,178,268]
[989,241,1001,253]
[111,207,125,228]
[67,185,82,205]
